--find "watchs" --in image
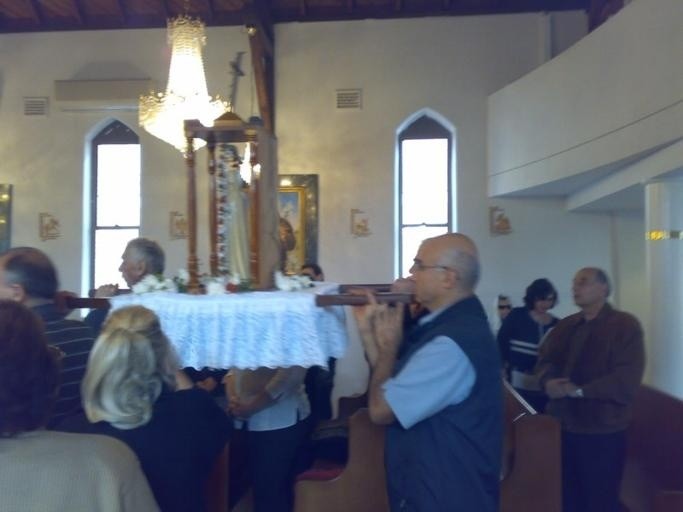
[577,388,584,399]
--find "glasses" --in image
[415,263,459,279]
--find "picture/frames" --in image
[276,172,320,276]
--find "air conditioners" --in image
[51,79,158,110]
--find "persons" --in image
[534,266,645,512]
[74,303,232,512]
[496,276,562,412]
[352,233,504,512]
[0,246,102,428]
[225,365,317,512]
[93,239,178,296]
[0,296,161,512]
[301,263,335,435]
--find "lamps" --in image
[645,230,683,241]
[137,10,233,159]
